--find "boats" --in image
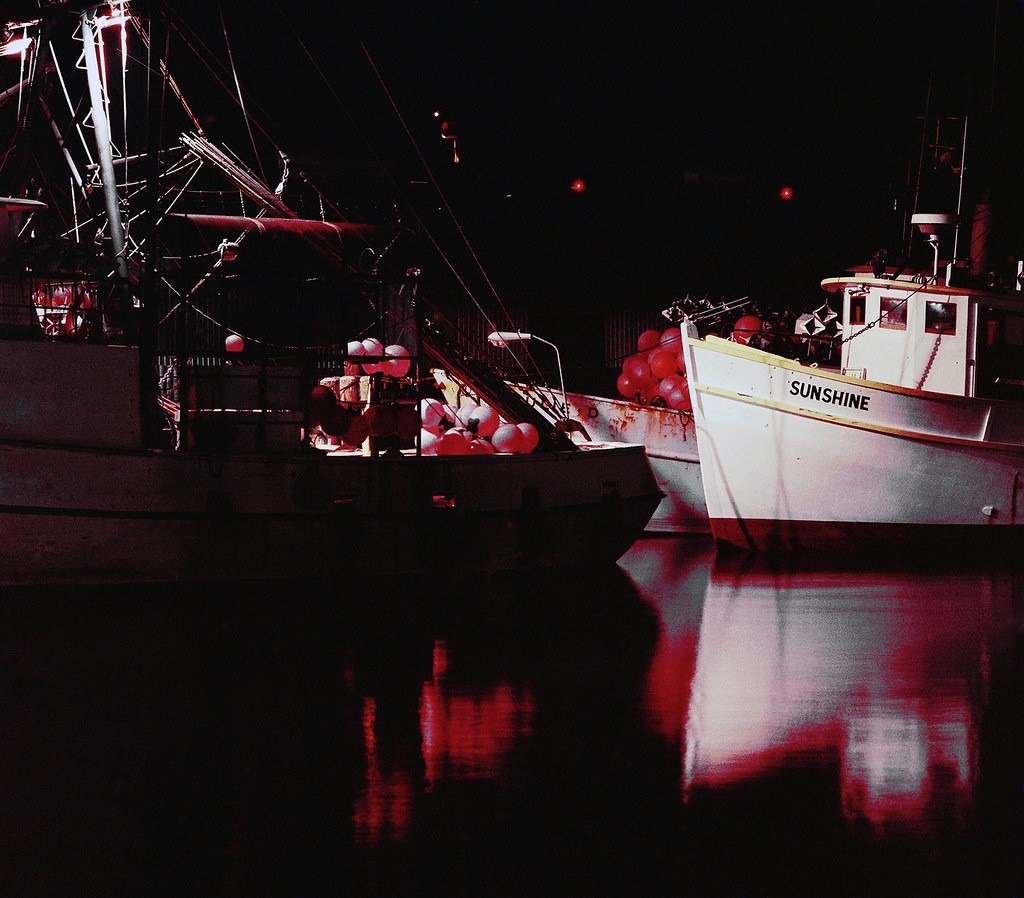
[680,92,1023,571]
[432,298,710,538]
[0,0,662,636]
